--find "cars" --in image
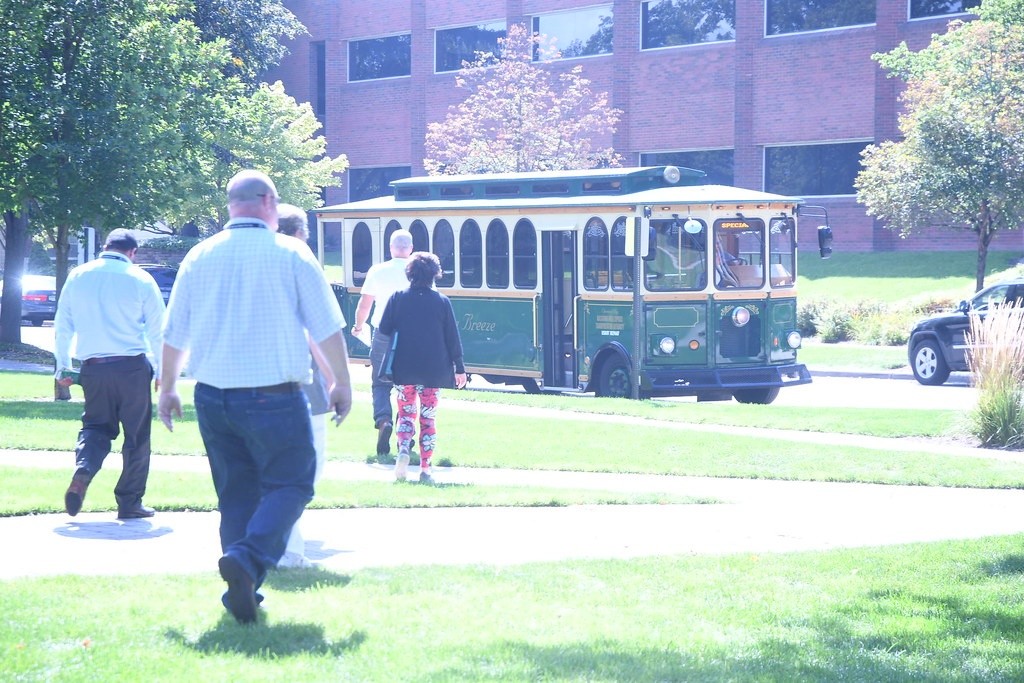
[908,281,1024,386]
[0,275,56,327]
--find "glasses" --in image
[257,194,280,205]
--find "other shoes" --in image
[376,420,393,455]
[394,454,410,481]
[419,473,430,482]
[221,591,265,612]
[398,437,416,452]
[217,553,259,624]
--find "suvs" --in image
[132,263,178,309]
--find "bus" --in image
[304,165,835,405]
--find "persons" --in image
[162,169,353,622]
[54,228,167,518]
[693,238,739,288]
[274,202,335,388]
[354,229,435,454]
[372,251,467,484]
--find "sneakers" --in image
[118,504,155,518]
[66,483,83,517]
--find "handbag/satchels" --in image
[378,289,403,382]
